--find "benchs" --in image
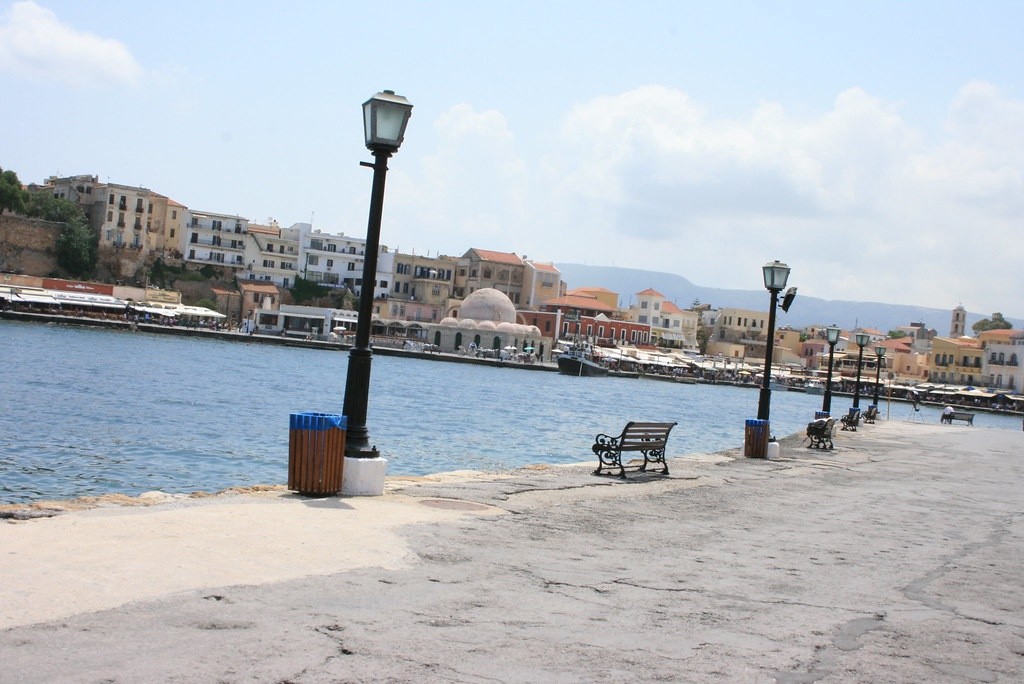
[944,412,976,427]
[861,405,880,424]
[840,408,860,431]
[806,418,839,450]
[591,421,678,479]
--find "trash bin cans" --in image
[849,408,860,426]
[815,412,830,438]
[288,412,347,498]
[868,405,877,419]
[744,419,770,458]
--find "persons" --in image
[940,404,954,424]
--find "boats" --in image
[804,380,825,395]
[557,350,609,377]
[608,369,641,379]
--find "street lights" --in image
[873,346,886,405]
[853,332,870,408]
[340,90,415,458]
[756,261,798,441]
[823,324,841,412]
